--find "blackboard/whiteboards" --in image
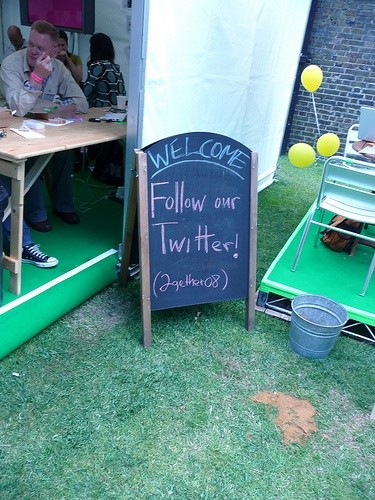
[122,132,258,311]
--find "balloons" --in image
[300,65,323,92]
[288,143,316,168]
[316,132,340,157]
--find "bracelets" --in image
[30,72,43,84]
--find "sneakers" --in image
[21,243,58,267]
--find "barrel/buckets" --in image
[288,294,349,361]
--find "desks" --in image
[0,105,127,294]
[351,140,375,160]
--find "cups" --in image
[116,96,127,109]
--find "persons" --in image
[55,30,83,83]
[1,105,58,268]
[1,20,90,232]
[82,33,126,183]
[0,25,28,66]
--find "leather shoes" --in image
[27,220,52,232]
[56,210,79,225]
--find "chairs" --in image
[343,123,361,158]
[291,155,375,296]
